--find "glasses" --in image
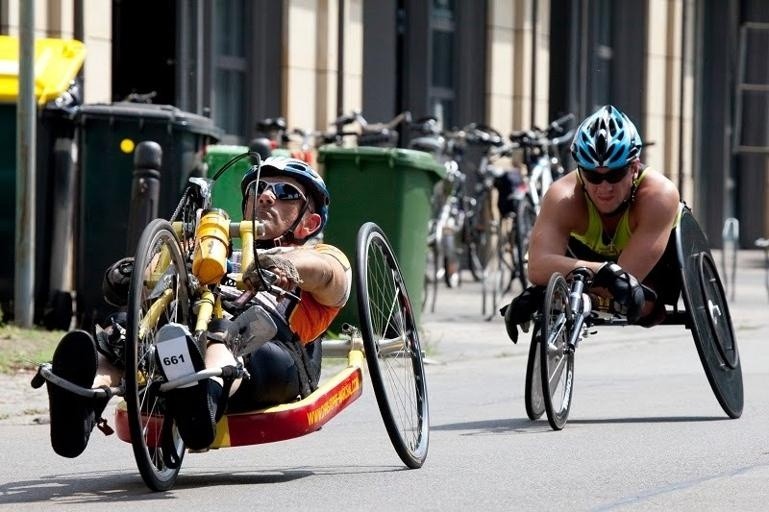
[580,163,631,183]
[245,180,313,212]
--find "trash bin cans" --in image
[2,35,86,313]
[205,143,292,252]
[78,101,222,327]
[317,146,446,340]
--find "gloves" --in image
[504,286,545,345]
[595,261,644,325]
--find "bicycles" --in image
[256,110,577,320]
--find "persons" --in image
[49,159,353,458]
[501,105,680,342]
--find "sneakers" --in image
[155,324,216,450]
[49,330,98,458]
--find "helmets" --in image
[571,104,642,169]
[241,158,330,239]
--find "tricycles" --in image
[37,186,429,494]
[523,199,744,431]
